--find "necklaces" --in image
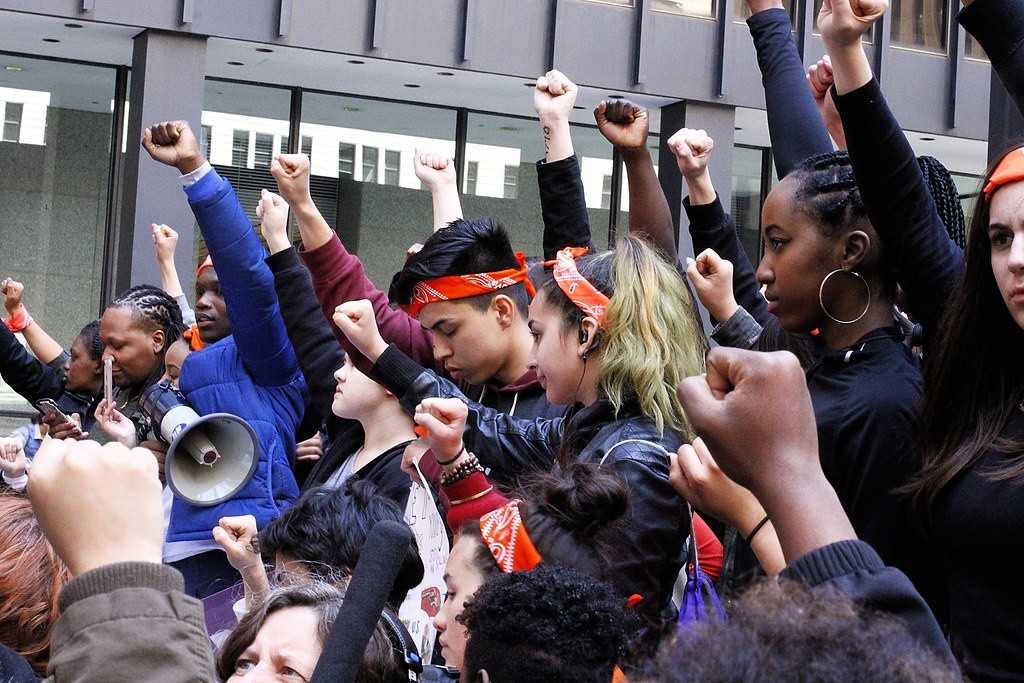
[803,334,898,374]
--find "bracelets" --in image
[440,451,482,487]
[452,482,493,507]
[437,441,465,466]
[747,515,770,543]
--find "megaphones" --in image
[138,378,259,507]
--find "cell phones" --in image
[36,398,84,435]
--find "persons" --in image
[0,0,1024,683]
